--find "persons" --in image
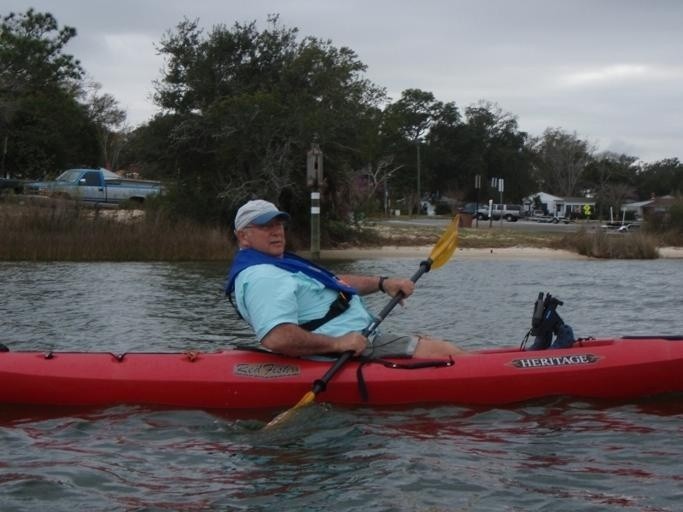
[224,199,463,359]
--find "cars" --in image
[616,223,642,233]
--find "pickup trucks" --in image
[478,205,525,222]
[22,169,159,203]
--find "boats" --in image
[0,336,682,409]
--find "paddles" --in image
[264,215,460,425]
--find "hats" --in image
[235,199,289,231]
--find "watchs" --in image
[378,274,389,293]
